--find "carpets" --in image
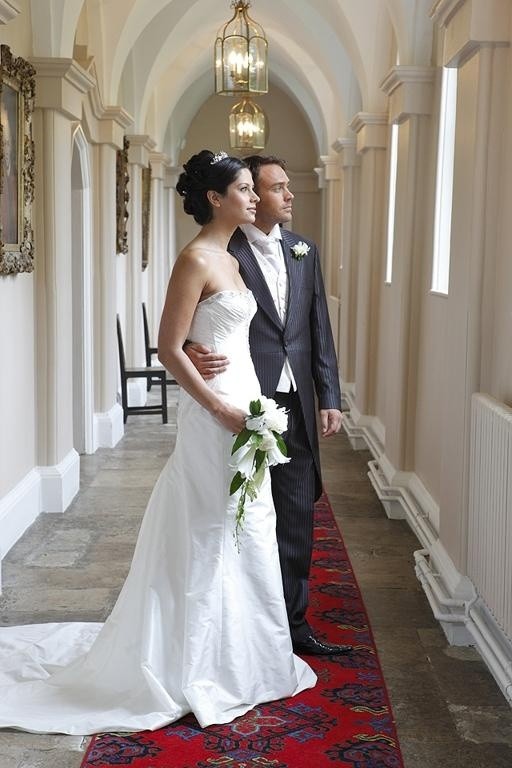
[79,483,404,767]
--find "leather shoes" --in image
[293,635,341,655]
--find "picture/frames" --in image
[114,133,157,273]
[0,46,38,281]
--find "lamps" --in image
[211,0,273,157]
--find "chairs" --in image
[116,300,179,426]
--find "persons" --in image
[86,151,320,728]
[180,154,355,659]
